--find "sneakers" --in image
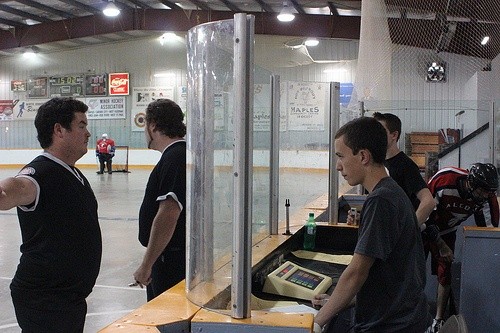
[431,318,444,333]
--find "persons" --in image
[311,117,432,332]
[417,162,500,325]
[134,98,186,301]
[96,134,116,175]
[0,98,102,333]
[369,112,435,229]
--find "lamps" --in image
[306,37,319,46]
[276,1,296,22]
[104,1,119,16]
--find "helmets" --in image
[102,133,107,137]
[470,161,499,192]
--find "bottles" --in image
[303,213,316,250]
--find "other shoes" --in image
[109,170,112,174]
[95,171,103,174]
